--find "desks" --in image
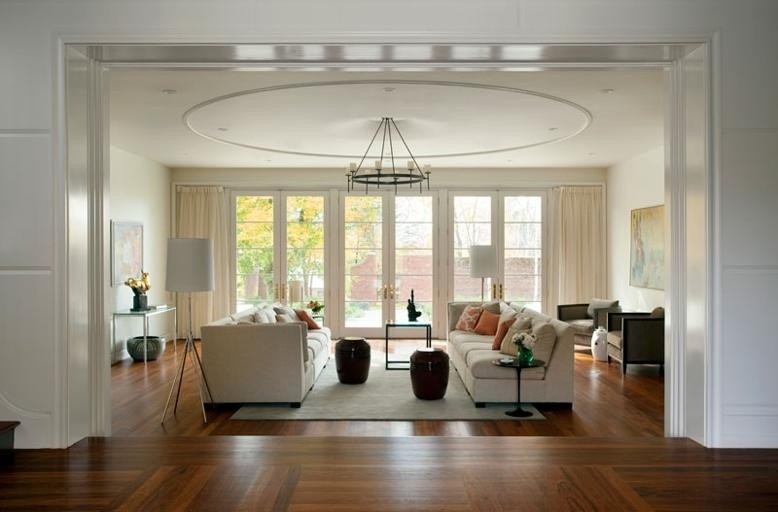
[491,359,545,417]
[386,320,431,371]
[113,307,176,365]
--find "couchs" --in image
[607,312,664,376]
[447,302,574,410]
[201,303,331,408]
[557,303,621,346]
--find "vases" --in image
[518,345,534,367]
[133,296,147,310]
[312,309,320,315]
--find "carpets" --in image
[230,353,546,420]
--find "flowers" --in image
[306,301,325,308]
[124,271,152,295]
[511,332,537,350]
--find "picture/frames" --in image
[110,220,144,287]
[629,205,665,291]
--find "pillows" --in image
[265,302,282,308]
[587,298,619,318]
[276,313,292,322]
[481,302,499,314]
[293,308,321,329]
[456,305,482,332]
[473,310,501,336]
[513,313,533,319]
[649,307,664,318]
[239,315,254,321]
[499,302,518,321]
[492,317,516,350]
[500,328,529,355]
[510,316,533,330]
[273,306,301,321]
[256,308,277,323]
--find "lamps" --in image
[470,246,497,302]
[345,117,431,196]
[161,238,215,425]
